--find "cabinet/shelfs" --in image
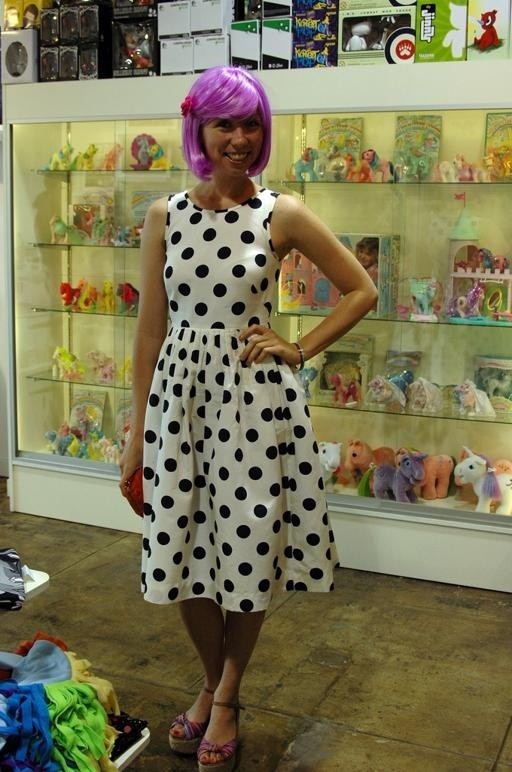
[2,59,512,593]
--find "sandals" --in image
[197,696,245,772]
[168,686,214,754]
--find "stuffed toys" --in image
[319,438,511,517]
[42,404,131,461]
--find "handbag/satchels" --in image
[0,548,25,611]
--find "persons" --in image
[356,237,378,310]
[117,66,379,771]
